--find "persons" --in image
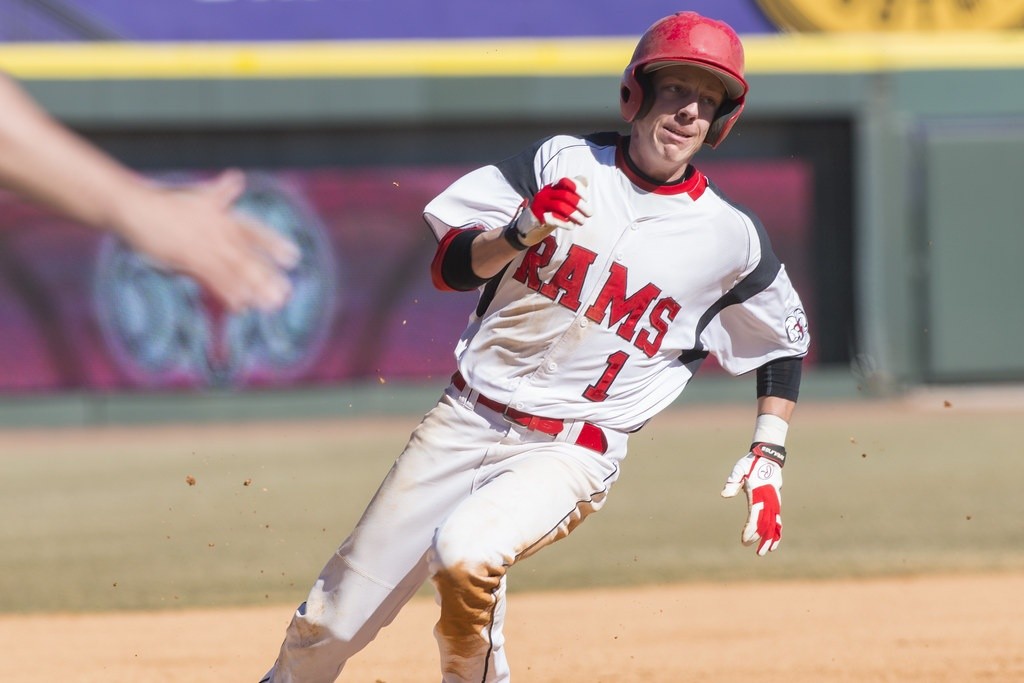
[0,71,301,316]
[258,9,810,682]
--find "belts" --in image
[452,372,607,455]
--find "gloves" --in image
[721,442,786,556]
[506,177,593,251]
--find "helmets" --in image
[619,11,749,149]
